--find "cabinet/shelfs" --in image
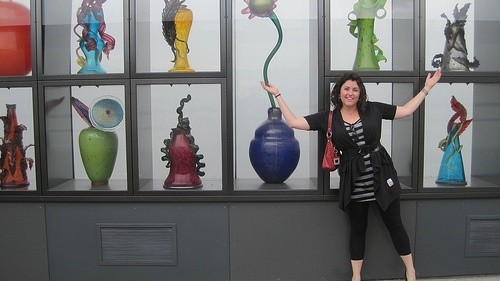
[0,0,229,197]
[327,1,500,195]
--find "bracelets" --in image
[275,93,281,98]
[422,88,428,96]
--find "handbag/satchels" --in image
[322,111,340,171]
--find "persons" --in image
[259,67,442,281]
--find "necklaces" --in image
[341,107,357,116]
